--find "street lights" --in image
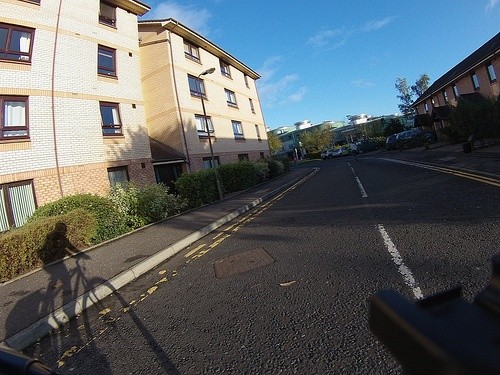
[193,66,228,204]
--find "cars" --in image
[397,129,434,146]
[319,136,384,162]
[386,132,402,150]
